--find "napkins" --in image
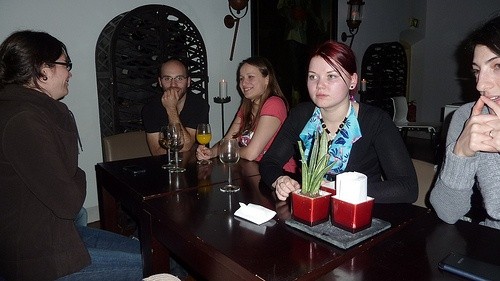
[336,172,368,204]
[234,202,277,224]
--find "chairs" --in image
[390,96,436,148]
[413,159,438,209]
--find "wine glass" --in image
[158,127,187,173]
[218,138,241,193]
[195,123,213,166]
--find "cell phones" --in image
[123,165,145,174]
[438,252,500,281]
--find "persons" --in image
[258,41,418,204]
[143,57,210,156]
[429,16,500,229]
[195,56,302,174]
[0,30,144,281]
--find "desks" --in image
[143,174,431,281]
[95,151,260,258]
[311,211,500,281]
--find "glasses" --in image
[40,60,72,73]
[160,75,189,83]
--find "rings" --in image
[279,182,284,186]
[488,130,494,138]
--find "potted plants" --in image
[290,132,332,228]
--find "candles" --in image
[218,79,228,100]
[361,78,368,92]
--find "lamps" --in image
[342,0,365,48]
[224,0,248,61]
